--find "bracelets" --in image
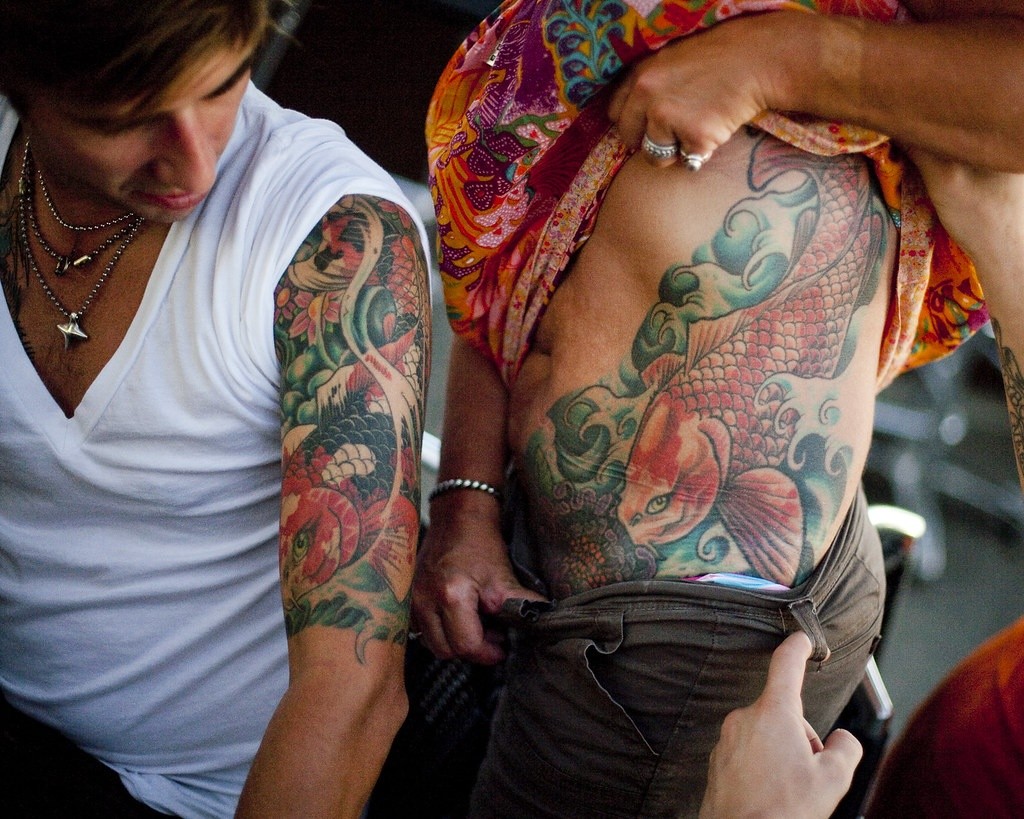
[428,478,500,502]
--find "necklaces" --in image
[15,135,143,351]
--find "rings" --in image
[641,133,678,160]
[680,148,712,172]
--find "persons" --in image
[412,0,1024,819]
[1,0,432,819]
